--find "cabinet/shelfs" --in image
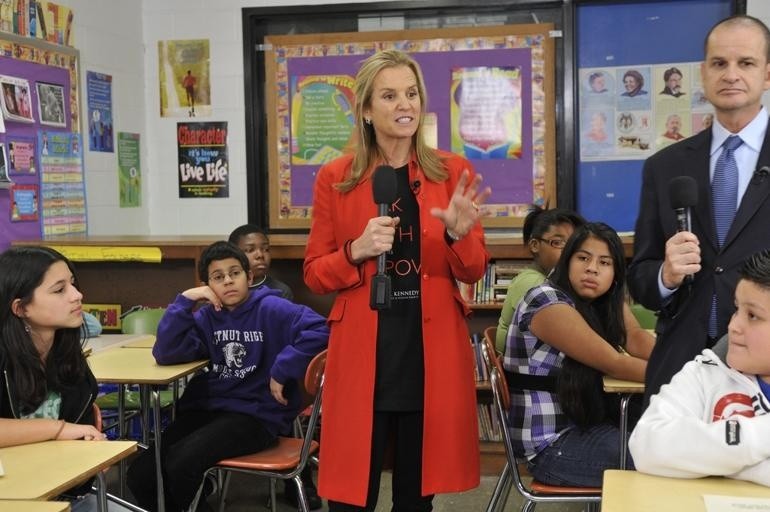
[467,304,507,454]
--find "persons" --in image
[182,70,198,107]
[628,247,769,489]
[585,115,609,141]
[616,71,648,97]
[126,240,332,511]
[503,223,658,488]
[666,114,685,141]
[588,70,612,94]
[626,12,770,413]
[658,68,688,98]
[0,245,108,501]
[228,224,294,305]
[4,83,65,121]
[495,196,584,353]
[300,49,494,512]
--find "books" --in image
[454,260,527,443]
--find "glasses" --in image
[531,235,569,249]
[208,269,248,283]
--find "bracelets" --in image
[345,238,364,267]
[53,419,68,440]
[447,229,463,241]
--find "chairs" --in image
[489,354,603,512]
[481,327,526,512]
[629,304,660,328]
[94,309,174,439]
[214,348,329,512]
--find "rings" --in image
[473,202,479,211]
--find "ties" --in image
[703,132,746,339]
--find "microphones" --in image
[368,164,397,310]
[667,173,701,287]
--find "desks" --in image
[604,329,657,470]
[602,469,770,512]
[0,334,213,512]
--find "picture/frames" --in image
[242,0,579,234]
